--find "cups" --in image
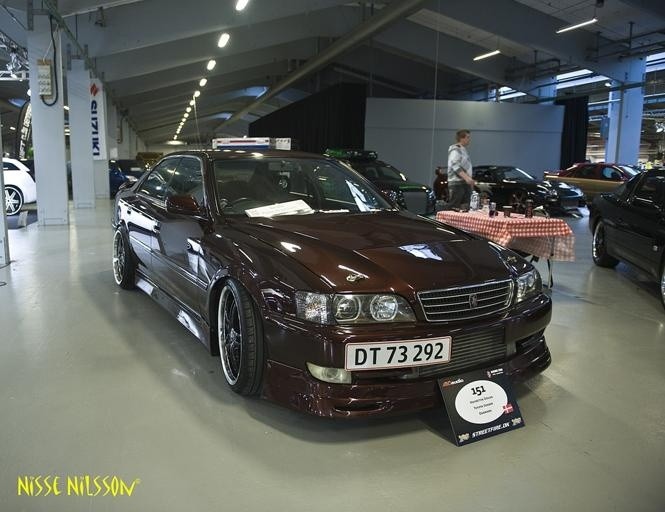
[503,205,512,217]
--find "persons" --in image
[449,129,475,211]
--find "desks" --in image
[436,209,574,289]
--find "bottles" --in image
[470,191,498,218]
[526,196,534,218]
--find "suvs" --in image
[309,149,438,220]
[134,152,163,171]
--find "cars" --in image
[431,162,588,216]
[110,149,554,426]
[1,156,38,215]
[585,154,605,163]
[584,168,665,313]
[544,158,645,205]
[268,154,302,193]
[65,160,145,195]
